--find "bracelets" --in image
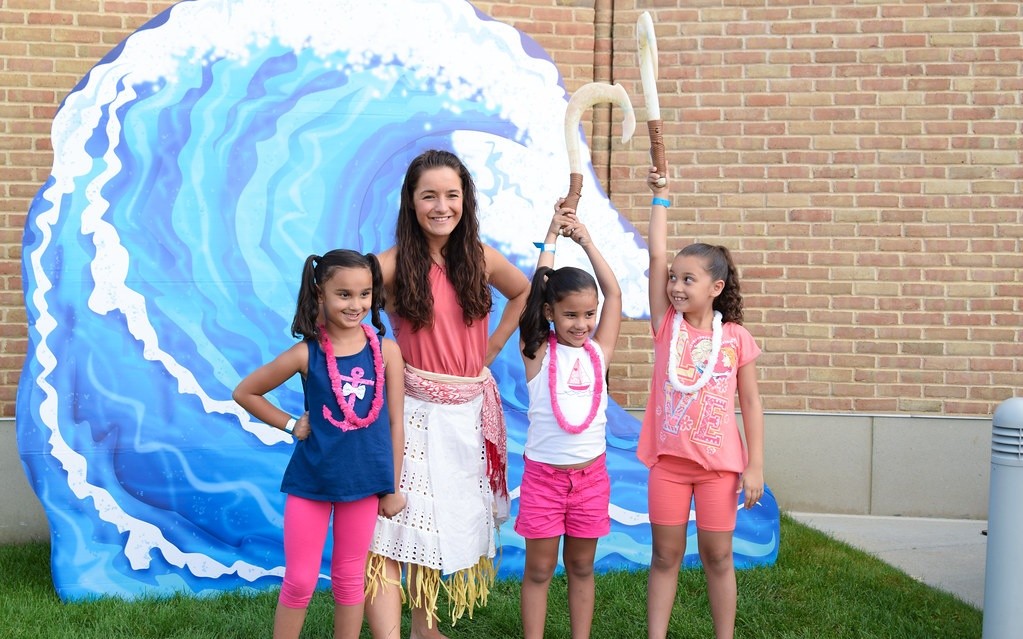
[653,197,670,208]
[533,242,556,255]
[284,416,298,434]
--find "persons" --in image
[636,159,764,639]
[231,249,407,639]
[315,150,533,639]
[514,198,622,639]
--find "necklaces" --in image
[668,311,722,394]
[317,321,385,427]
[546,330,603,434]
